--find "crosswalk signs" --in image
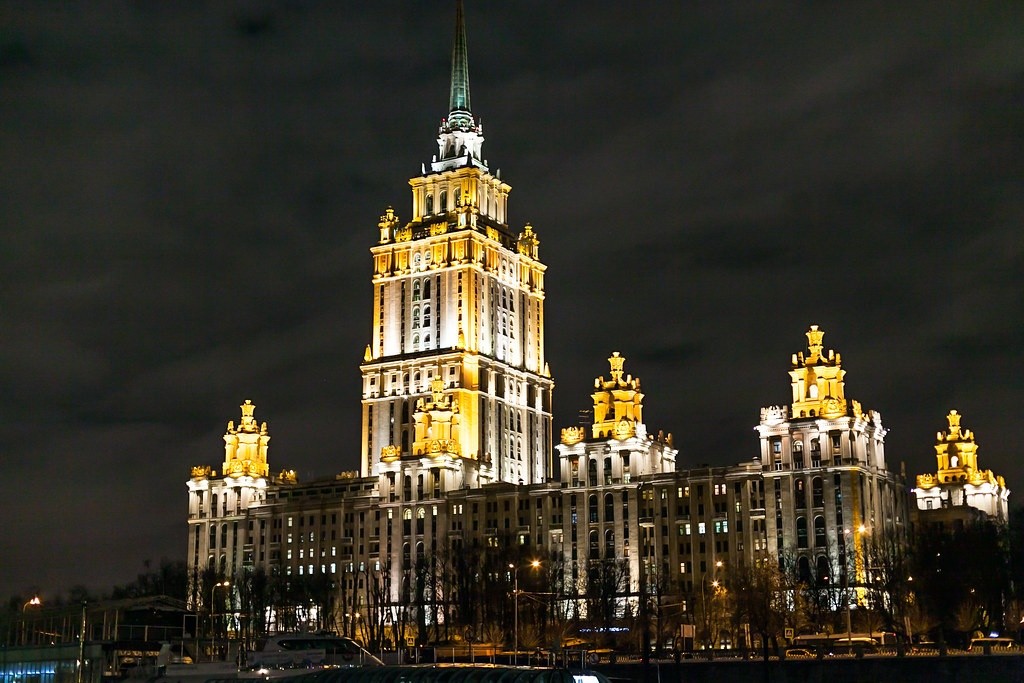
[785,628,793,640]
[407,638,414,646]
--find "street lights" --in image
[211,582,230,662]
[22,600,40,647]
[845,527,865,655]
[702,561,722,619]
[514,562,539,667]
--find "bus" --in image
[792,632,896,645]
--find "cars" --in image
[785,649,817,657]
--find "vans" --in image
[264,630,385,667]
[968,638,1023,651]
